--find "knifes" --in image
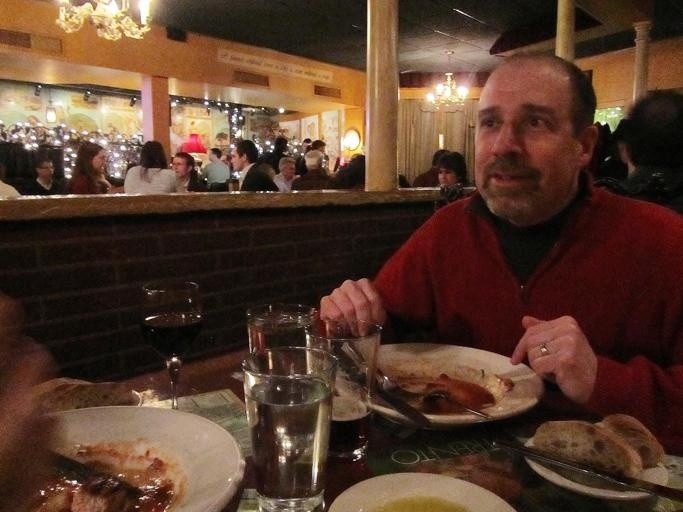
[332,347,433,432]
[493,440,683,501]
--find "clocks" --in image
[343,128,361,151]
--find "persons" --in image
[320,49,682,456]
[1,142,112,199]
[593,89,682,213]
[230,138,366,191]
[124,132,230,195]
[398,150,470,188]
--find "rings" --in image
[539,343,549,357]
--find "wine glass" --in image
[143,274,202,410]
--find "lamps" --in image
[57,0,153,42]
[426,51,468,106]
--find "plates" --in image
[524,436,670,500]
[370,343,544,427]
[327,472,518,512]
[22,378,142,405]
[23,405,246,511]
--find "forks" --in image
[347,342,422,398]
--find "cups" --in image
[240,300,382,512]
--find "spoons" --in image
[423,389,493,420]
